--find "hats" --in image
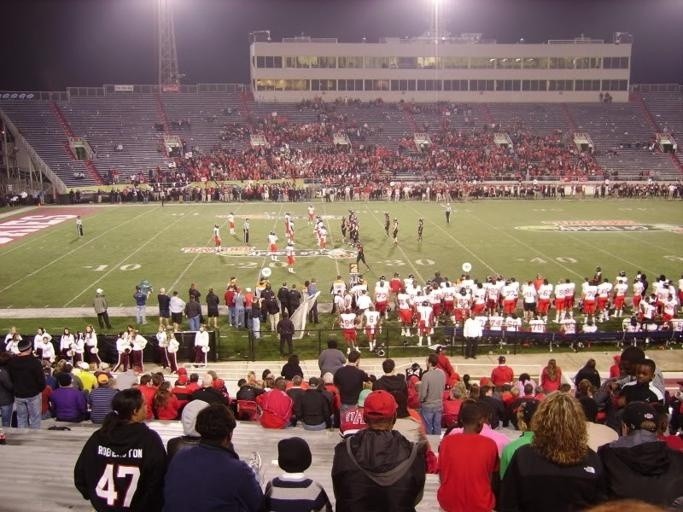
[78,362,89,369]
[182,399,210,438]
[98,374,109,384]
[615,402,658,433]
[309,377,319,387]
[278,437,310,472]
[175,368,187,375]
[96,288,104,293]
[363,390,399,417]
[323,372,334,384]
[18,339,32,351]
[357,389,373,407]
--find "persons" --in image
[0,352,14,427]
[184,295,201,331]
[284,212,290,237]
[5,332,23,355]
[436,400,498,510]
[338,389,373,441]
[417,401,463,435]
[67,97,683,205]
[317,339,345,378]
[92,288,113,330]
[205,288,219,327]
[73,388,167,512]
[40,323,73,423]
[439,203,452,224]
[213,224,223,252]
[267,232,272,251]
[448,400,510,458]
[499,400,542,480]
[313,216,328,249]
[270,232,280,262]
[578,401,619,434]
[333,351,369,412]
[331,390,426,510]
[307,204,314,225]
[578,397,619,453]
[649,401,682,454]
[326,271,390,350]
[131,285,149,324]
[486,262,682,345]
[361,375,376,392]
[226,402,340,430]
[496,390,607,510]
[169,291,185,332]
[383,212,389,239]
[390,268,486,346]
[287,220,295,244]
[224,272,318,369]
[596,400,682,510]
[0,190,46,208]
[489,400,542,432]
[72,325,264,403]
[243,218,251,246]
[355,243,370,273]
[157,287,172,330]
[392,218,399,243]
[407,346,683,401]
[416,217,423,242]
[73,403,186,423]
[188,284,206,323]
[3,326,22,343]
[284,240,298,273]
[9,339,46,429]
[75,216,84,238]
[163,402,264,511]
[264,369,339,402]
[372,359,408,405]
[261,436,331,511]
[340,208,360,249]
[166,399,212,464]
[661,401,683,436]
[390,388,417,443]
[226,212,235,234]
